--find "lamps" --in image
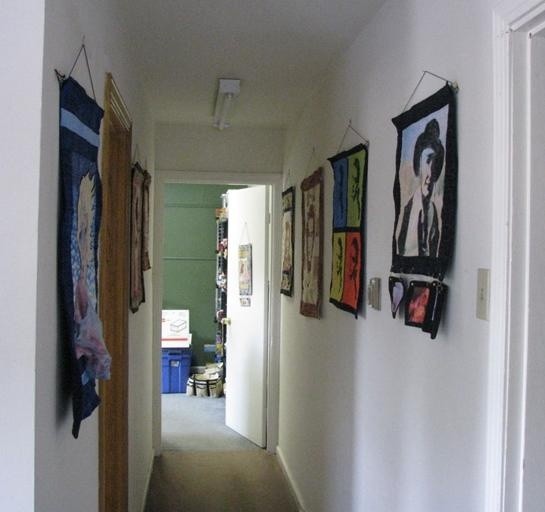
[210,78,242,130]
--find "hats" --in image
[414,119,444,181]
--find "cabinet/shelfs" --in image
[214,191,229,363]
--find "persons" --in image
[302,203,321,307]
[331,159,360,308]
[69,170,96,321]
[394,119,445,261]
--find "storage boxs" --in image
[159,347,192,394]
